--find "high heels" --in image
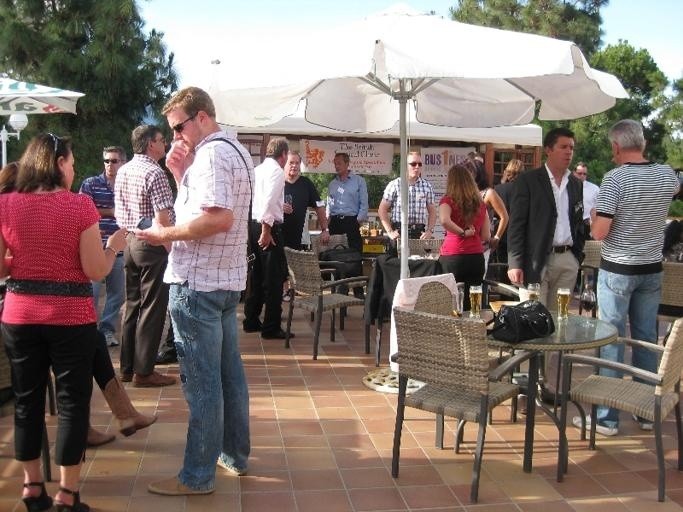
[21,480,53,512]
[51,486,92,512]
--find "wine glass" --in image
[580,290,597,329]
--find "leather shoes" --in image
[131,372,179,389]
[145,473,218,496]
[155,352,178,364]
[261,328,296,340]
[217,456,249,477]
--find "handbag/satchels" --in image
[244,221,257,305]
[485,299,556,345]
[319,242,364,281]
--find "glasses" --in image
[152,137,167,144]
[575,172,588,176]
[102,158,122,164]
[171,112,199,134]
[50,131,58,165]
[407,162,423,167]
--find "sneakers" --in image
[571,412,620,437]
[630,413,655,433]
[104,333,120,347]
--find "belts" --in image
[550,244,574,255]
[328,214,358,220]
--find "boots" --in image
[100,380,159,438]
[86,426,115,446]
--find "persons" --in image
[243,139,369,338]
[569,118,682,438]
[658,218,682,263]
[1,87,255,512]
[378,129,600,407]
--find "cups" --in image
[468,285,482,318]
[451,282,464,316]
[556,287,570,322]
[360,221,385,237]
[527,282,540,303]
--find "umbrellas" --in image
[0,74,88,120]
[199,11,632,279]
